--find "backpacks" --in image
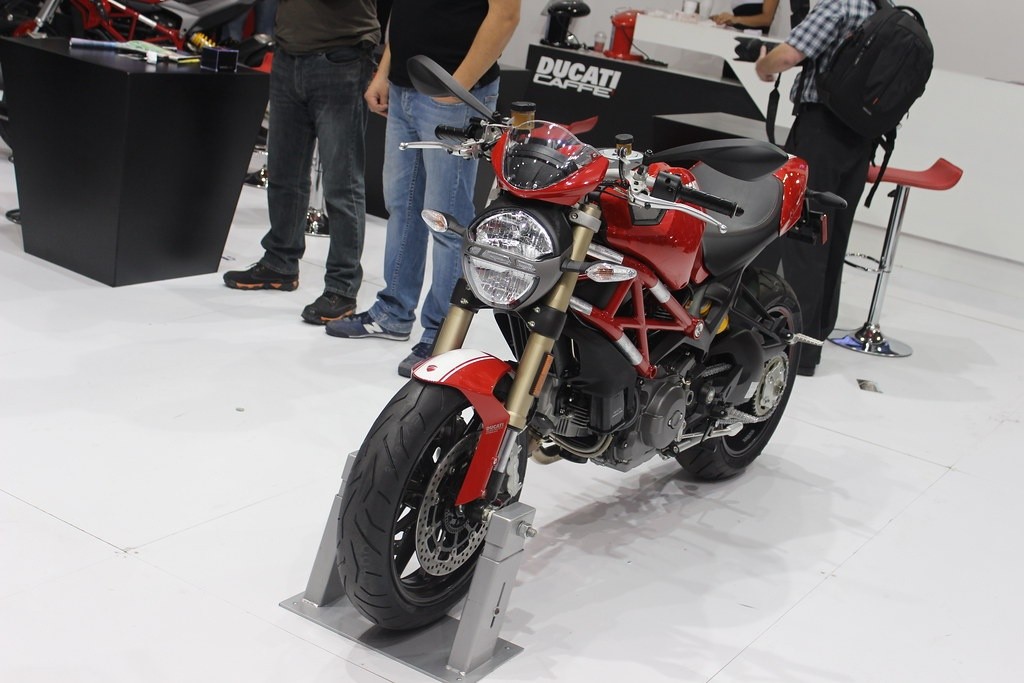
[815,0,934,142]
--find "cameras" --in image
[733,1,812,65]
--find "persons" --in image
[324,1,521,378]
[708,0,811,41]
[222,0,381,324]
[755,0,904,379]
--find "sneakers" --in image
[327,312,411,341]
[302,290,356,325]
[224,263,299,291]
[398,342,434,378]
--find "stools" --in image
[824,156,964,360]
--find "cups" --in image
[682,1,700,14]
[699,0,715,17]
[593,32,607,53]
[510,100,537,132]
[616,134,634,157]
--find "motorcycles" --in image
[1,0,280,146]
[335,52,848,633]
